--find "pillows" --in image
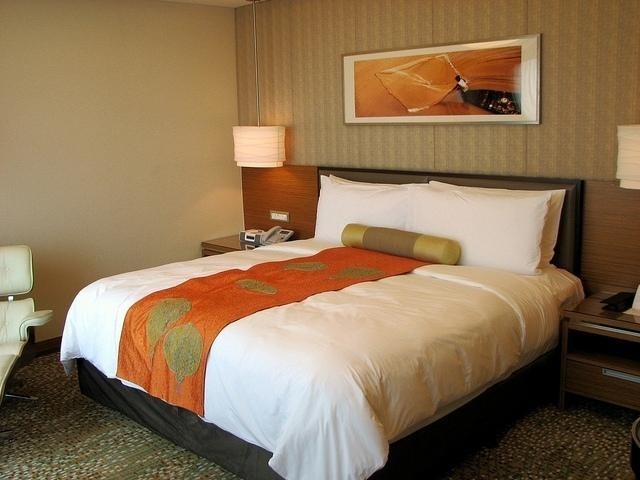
[308,170,568,277]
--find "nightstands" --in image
[199,231,264,257]
[550,286,639,421]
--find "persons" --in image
[451,75,472,103]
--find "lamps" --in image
[232,0,287,170]
[615,123,640,190]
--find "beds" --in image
[56,164,588,479]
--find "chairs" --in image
[1,243,54,405]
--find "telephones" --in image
[261,225,295,246]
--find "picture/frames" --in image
[340,33,542,126]
